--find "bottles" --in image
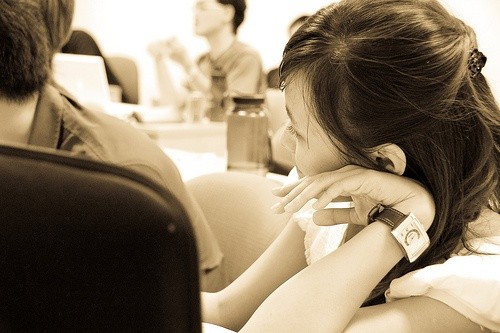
[225,95,270,174]
[210,67,227,122]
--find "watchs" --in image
[367,202,430,263]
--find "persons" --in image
[200,0,500,333]
[0,0,306,276]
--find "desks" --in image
[109,101,228,165]
